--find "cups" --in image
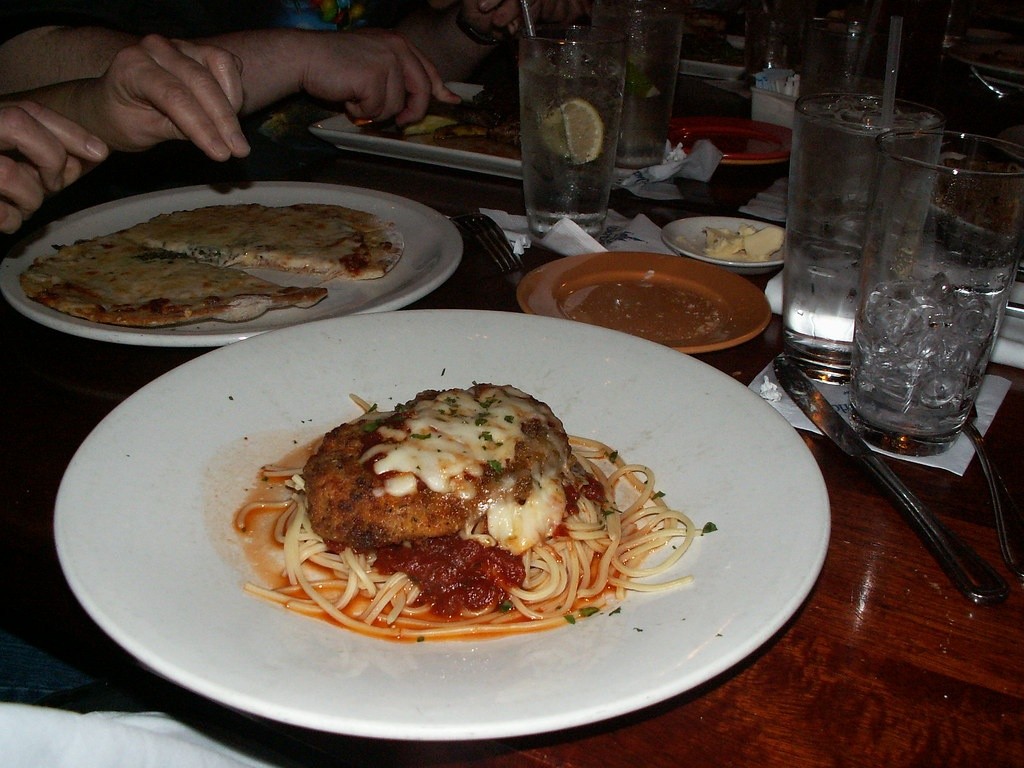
[800,18,875,99]
[745,10,788,89]
[783,94,947,371]
[848,130,1024,456]
[592,6,684,171]
[519,25,628,238]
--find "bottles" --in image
[930,54,971,124]
[921,0,962,90]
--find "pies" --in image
[20,203,406,327]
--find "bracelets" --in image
[457,13,497,46]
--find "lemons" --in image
[560,98,604,165]
[541,107,570,159]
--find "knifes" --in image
[773,357,1010,606]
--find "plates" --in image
[966,28,1012,40]
[998,126,1024,159]
[660,217,787,275]
[51,309,830,742]
[0,181,464,348]
[678,34,747,80]
[666,115,794,165]
[309,81,525,181]
[959,43,1024,75]
[516,252,771,354]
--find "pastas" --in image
[235,395,705,636]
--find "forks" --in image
[970,65,1018,100]
[441,213,522,272]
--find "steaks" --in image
[430,83,525,146]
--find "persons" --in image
[0,0,594,235]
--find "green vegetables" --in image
[360,367,721,625]
